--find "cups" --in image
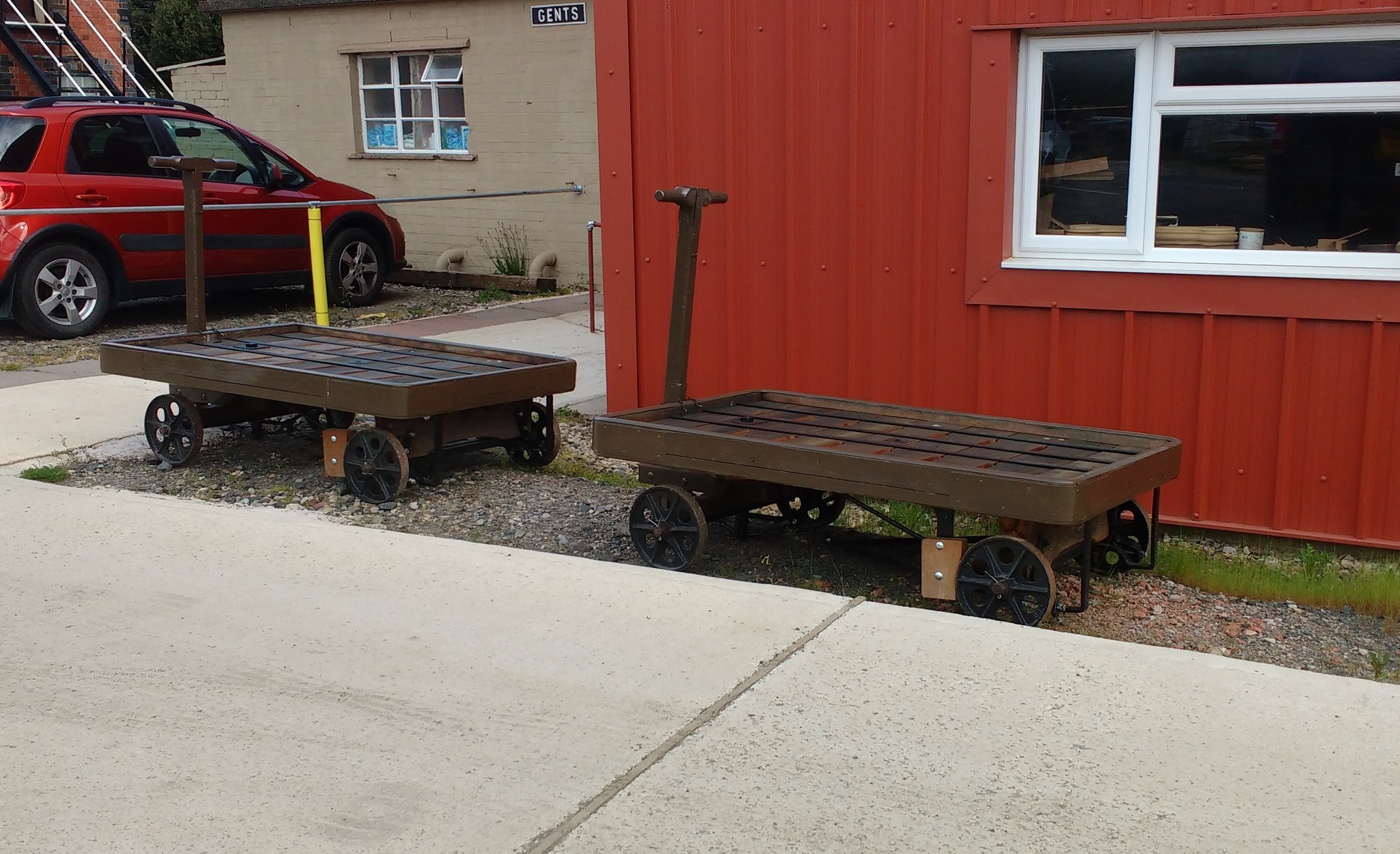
[1239,229,1265,250]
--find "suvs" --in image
[0,96,408,341]
